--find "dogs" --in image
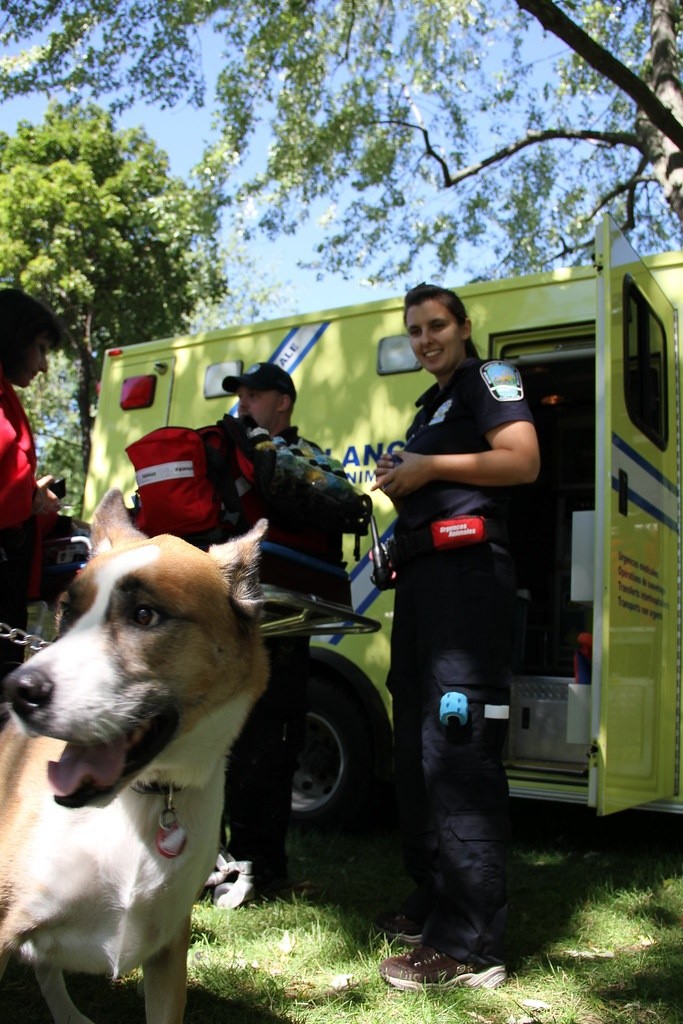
[0,491,271,1022]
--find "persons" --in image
[179,358,367,918]
[0,281,70,711]
[362,280,545,990]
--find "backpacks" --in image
[126,426,265,542]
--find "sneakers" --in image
[380,943,507,989]
[365,908,424,945]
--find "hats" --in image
[222,362,296,398]
